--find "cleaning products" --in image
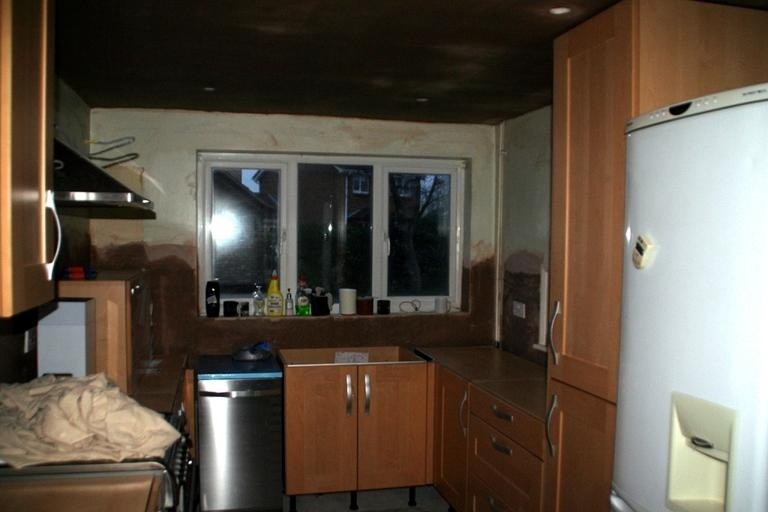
[266,270,283,316]
[295,275,311,316]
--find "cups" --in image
[223,301,239,317]
[338,288,391,316]
[434,297,450,314]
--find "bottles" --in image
[285,293,293,318]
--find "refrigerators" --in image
[607,81,767,510]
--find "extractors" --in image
[53,139,155,221]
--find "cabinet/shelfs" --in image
[0,0,65,319]
[283,363,432,496]
[550,0,768,512]
[415,342,545,512]
[58,269,153,395]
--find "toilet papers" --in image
[339,288,357,315]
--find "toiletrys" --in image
[252,285,265,317]
